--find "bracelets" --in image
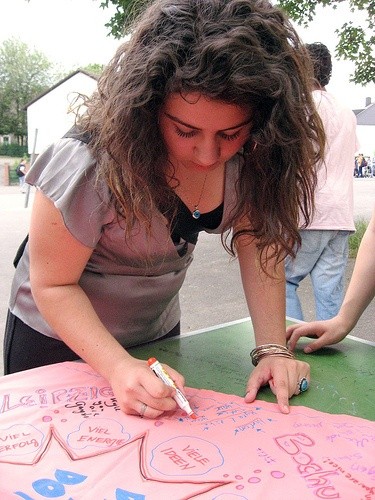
[249,345,297,367]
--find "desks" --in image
[0,317,375,500]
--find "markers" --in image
[147,357,197,420]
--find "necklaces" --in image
[175,172,208,219]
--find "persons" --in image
[286,202,375,358]
[18,158,29,190]
[4,0,327,419]
[354,153,375,178]
[287,41,360,322]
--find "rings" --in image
[296,377,307,391]
[140,404,147,416]
